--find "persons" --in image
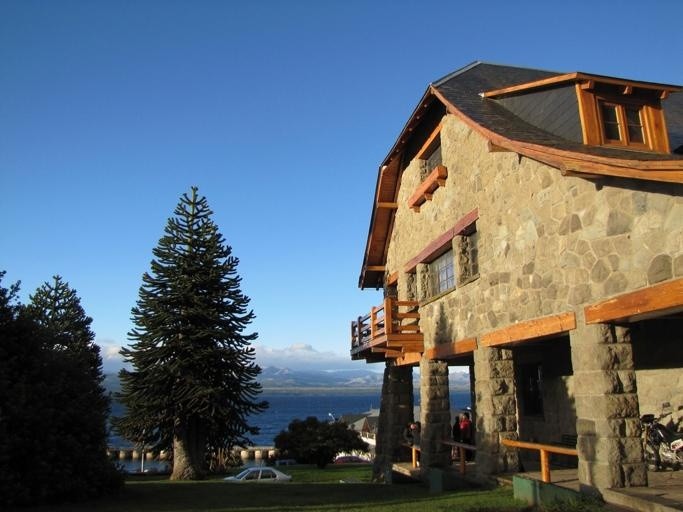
[459,411,474,463]
[404,422,415,461]
[450,415,460,462]
[410,419,422,467]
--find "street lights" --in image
[327,413,335,421]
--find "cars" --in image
[335,455,372,466]
[223,465,293,485]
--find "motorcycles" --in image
[639,402,682,474]
